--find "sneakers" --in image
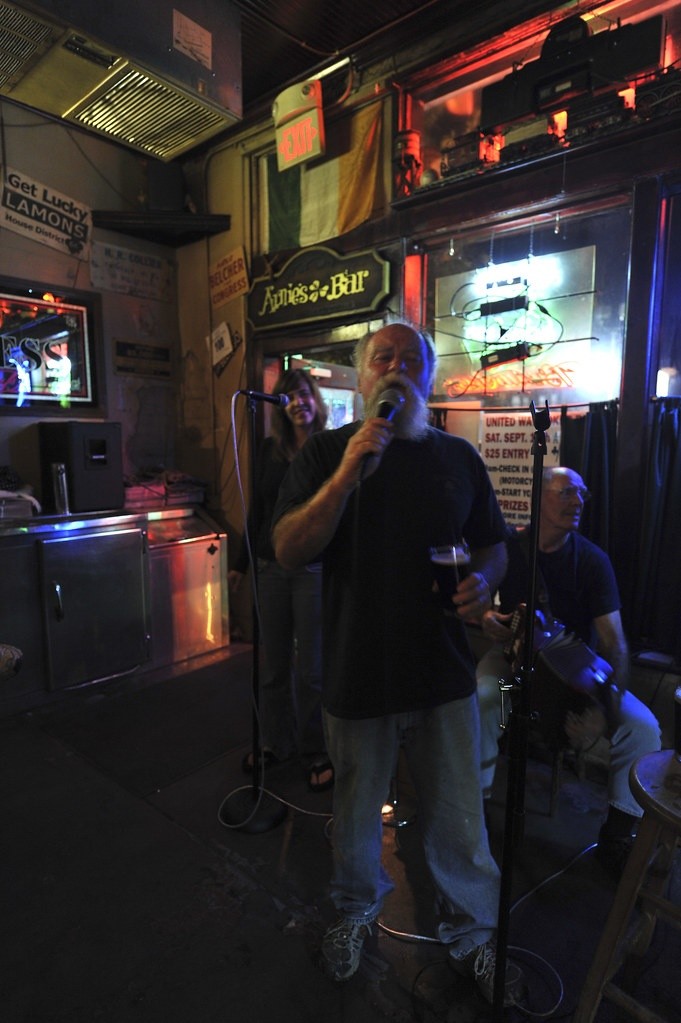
[318,916,368,983]
[449,940,527,1008]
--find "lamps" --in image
[271,80,323,128]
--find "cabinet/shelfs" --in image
[0,517,150,718]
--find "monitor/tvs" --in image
[0,274,109,419]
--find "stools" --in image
[576,751,681,1021]
[498,716,603,814]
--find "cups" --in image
[674,686,681,763]
[429,536,472,620]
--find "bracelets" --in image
[610,684,621,697]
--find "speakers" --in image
[37,422,125,513]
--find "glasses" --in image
[542,488,592,500]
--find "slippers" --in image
[242,749,273,774]
[309,761,334,793]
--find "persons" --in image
[475,468,663,883]
[271,323,525,1008]
[0,642,24,682]
[225,367,334,791]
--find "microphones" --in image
[241,385,294,412]
[356,388,405,481]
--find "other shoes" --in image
[1,644,23,680]
[598,827,638,852]
